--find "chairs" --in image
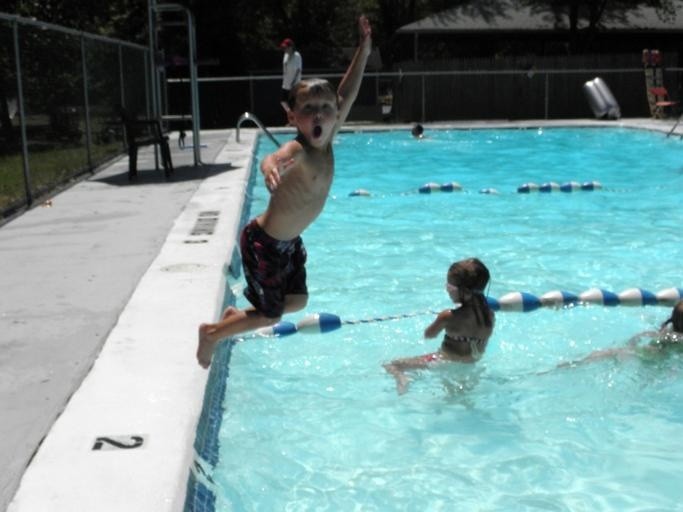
[119,108,174,180]
[649,79,683,141]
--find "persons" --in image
[197,13,374,371]
[410,122,426,140]
[551,302,682,371]
[380,255,495,394]
[278,39,302,126]
